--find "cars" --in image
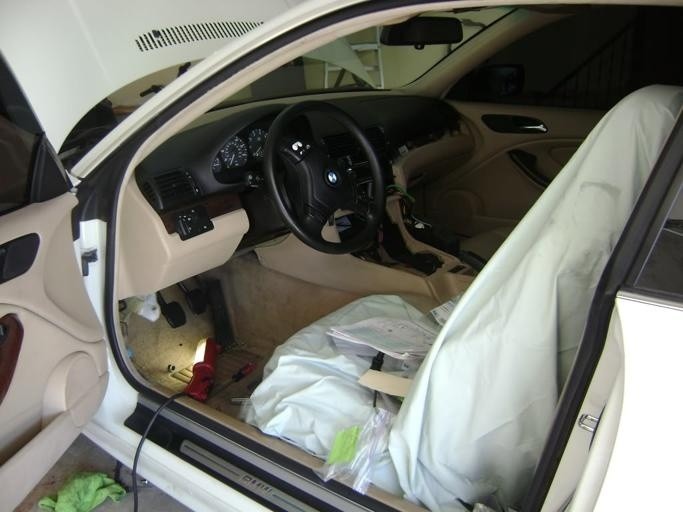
[0,2,681,512]
